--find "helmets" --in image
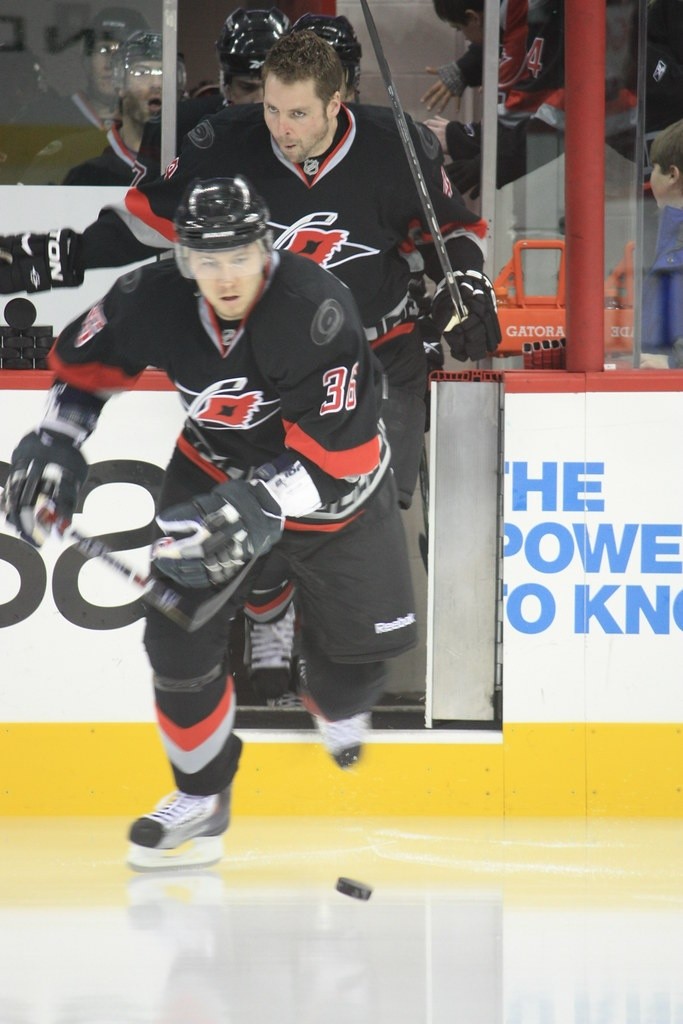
[110,29,187,89]
[173,175,273,280]
[94,9,148,41]
[290,12,362,93]
[215,7,291,74]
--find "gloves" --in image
[151,479,286,592]
[4,428,88,548]
[0,228,84,294]
[433,266,502,361]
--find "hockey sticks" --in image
[26,489,272,638]
[355,0,479,338]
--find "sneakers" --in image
[296,658,370,769]
[127,736,243,869]
[244,599,295,705]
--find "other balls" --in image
[332,875,377,904]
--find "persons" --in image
[0,24,506,704]
[56,0,683,203]
[637,120,683,371]
[0,172,418,852]
